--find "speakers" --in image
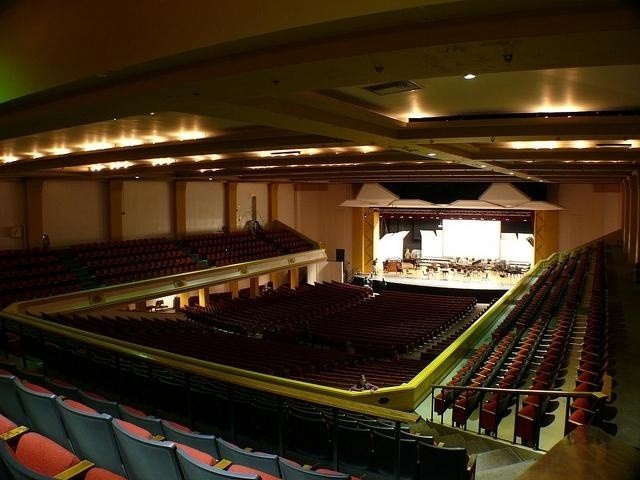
[336,249,344,261]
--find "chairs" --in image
[431,242,622,450]
[1,280,480,479]
[2,222,313,300]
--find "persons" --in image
[345,342,358,368]
[362,258,403,290]
[349,375,378,393]
[392,346,400,362]
